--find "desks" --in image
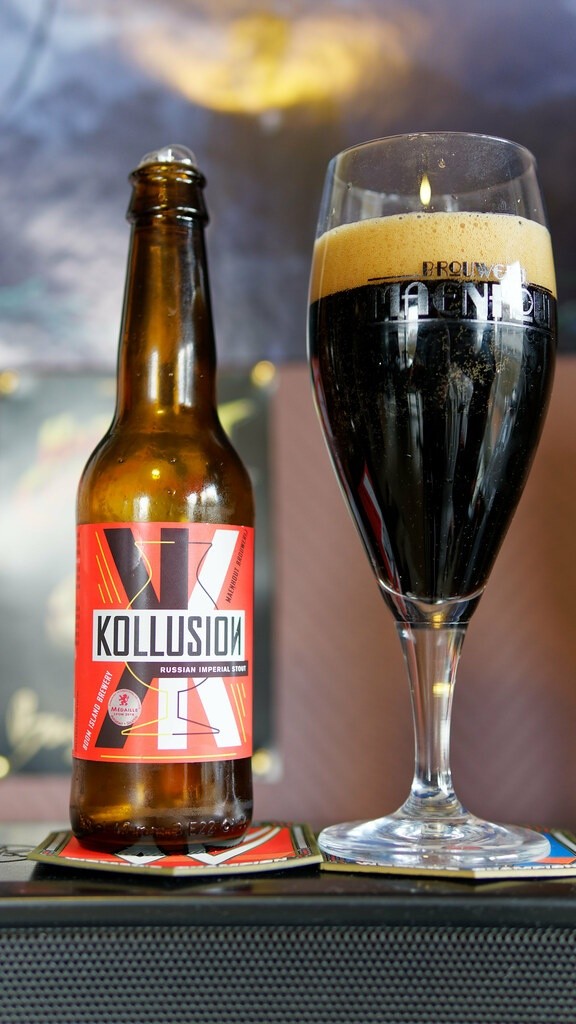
[2,821,576,1024]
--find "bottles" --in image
[68,158,264,852]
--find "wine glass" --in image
[302,128,562,867]
